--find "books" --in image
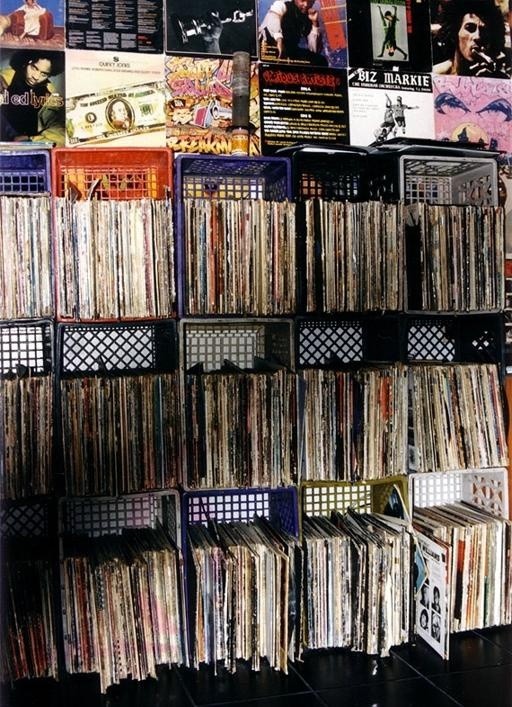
[2,362,512,695]
[3,190,501,320]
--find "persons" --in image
[258,0,328,67]
[432,0,511,78]
[191,9,223,55]
[0,49,66,148]
[374,94,418,143]
[376,5,407,60]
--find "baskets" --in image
[56,325,179,491]
[406,318,506,471]
[183,487,298,665]
[1,150,55,321]
[289,150,399,315]
[1,499,57,683]
[293,319,409,483]
[173,153,290,319]
[52,145,177,323]
[400,153,504,315]
[180,322,299,491]
[412,468,511,639]
[0,325,54,500]
[57,489,186,674]
[300,476,411,649]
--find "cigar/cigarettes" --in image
[479,51,494,64]
